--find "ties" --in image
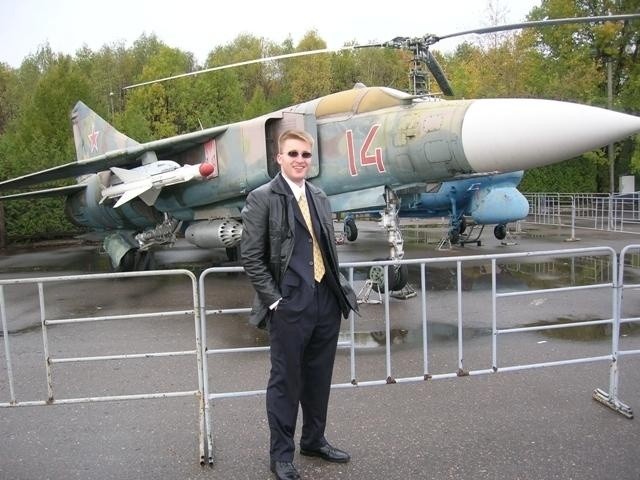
[297,192,326,284]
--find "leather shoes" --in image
[270,459,301,480]
[300,442,350,463]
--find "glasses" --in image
[283,150,313,158]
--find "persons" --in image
[237,129,363,480]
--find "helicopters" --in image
[121,13,640,244]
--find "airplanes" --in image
[0,83,640,294]
